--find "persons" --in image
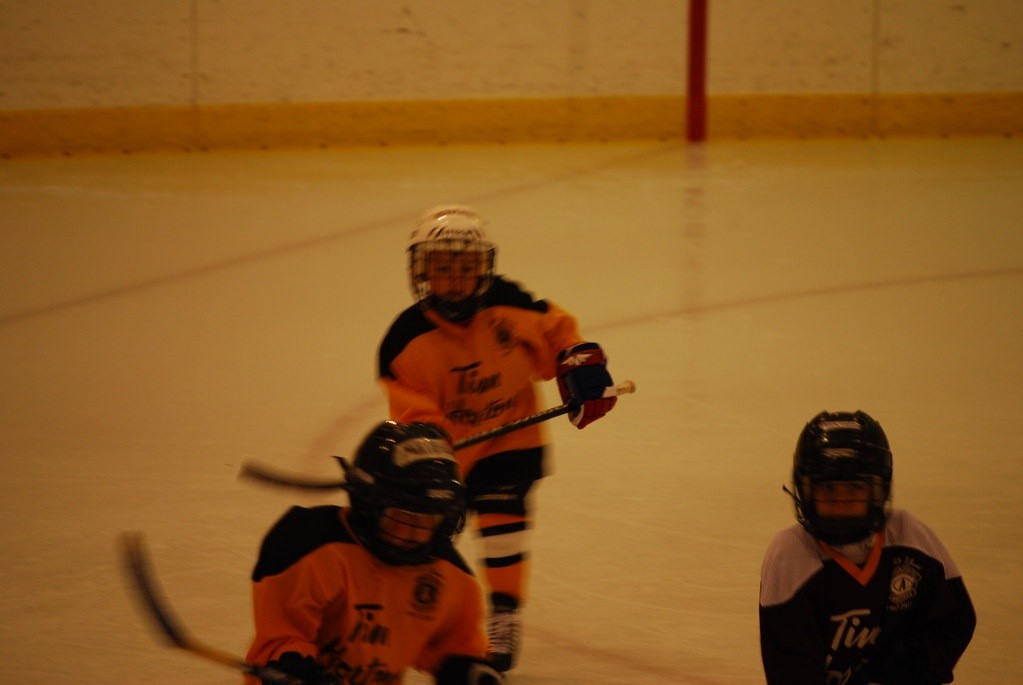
[373,211,616,673]
[241,420,507,685]
[759,409,976,685]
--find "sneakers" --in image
[480,592,527,674]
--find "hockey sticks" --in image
[110,526,390,685]
[237,379,637,496]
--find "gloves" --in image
[262,651,343,685]
[465,663,506,685]
[556,342,617,430]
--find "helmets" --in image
[344,420,475,566]
[407,205,497,305]
[792,410,893,543]
